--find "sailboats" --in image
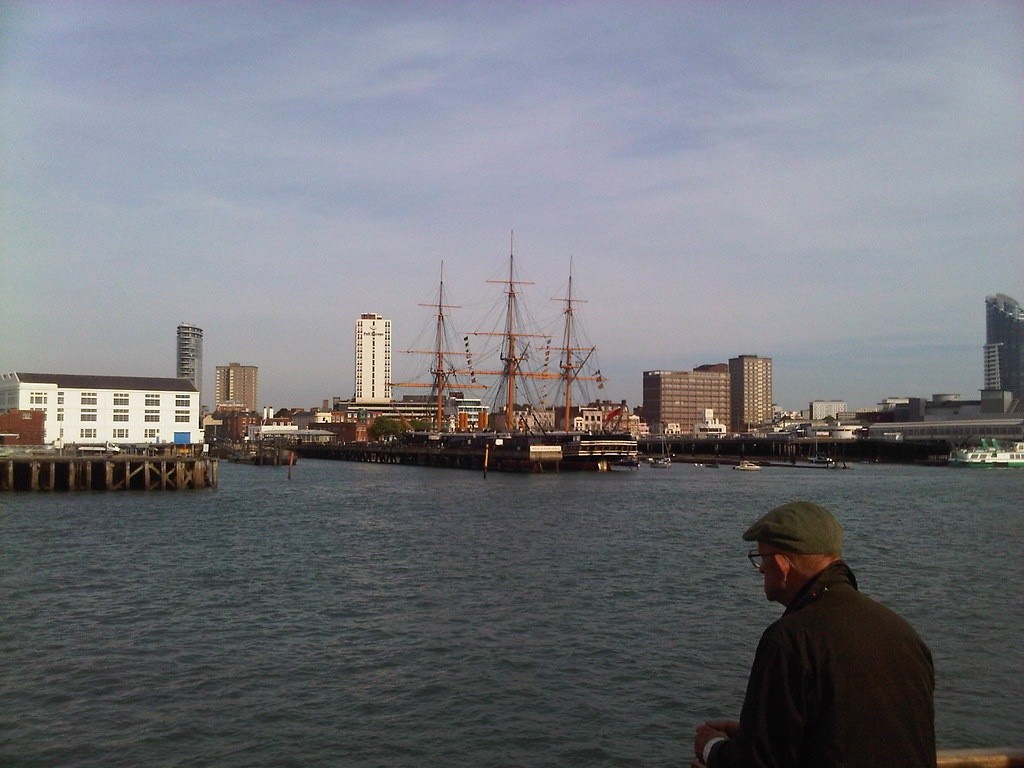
[650,421,671,468]
[370,229,640,473]
[807,417,833,463]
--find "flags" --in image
[607,408,621,419]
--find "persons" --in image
[169,442,174,455]
[694,501,936,768]
[518,418,524,430]
[512,419,516,429]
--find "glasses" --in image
[748,548,798,570]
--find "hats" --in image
[741,500,843,555]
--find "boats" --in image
[735,460,762,471]
[948,436,1023,470]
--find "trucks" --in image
[78,441,121,455]
